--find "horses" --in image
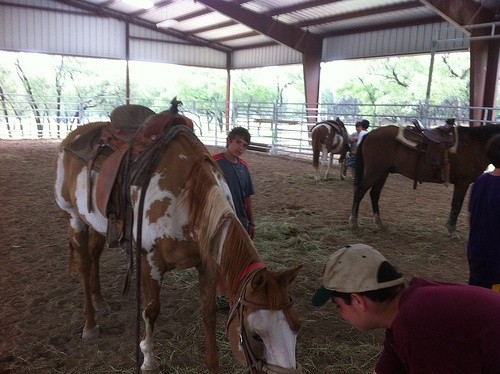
[310,117,348,181]
[350,118,500,238]
[54,96,305,374]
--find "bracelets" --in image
[248,222,255,227]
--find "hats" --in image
[311,243,404,307]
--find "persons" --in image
[348,120,370,180]
[210,126,256,315]
[466,134,500,290]
[311,243,500,374]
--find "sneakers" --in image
[216,293,230,314]
